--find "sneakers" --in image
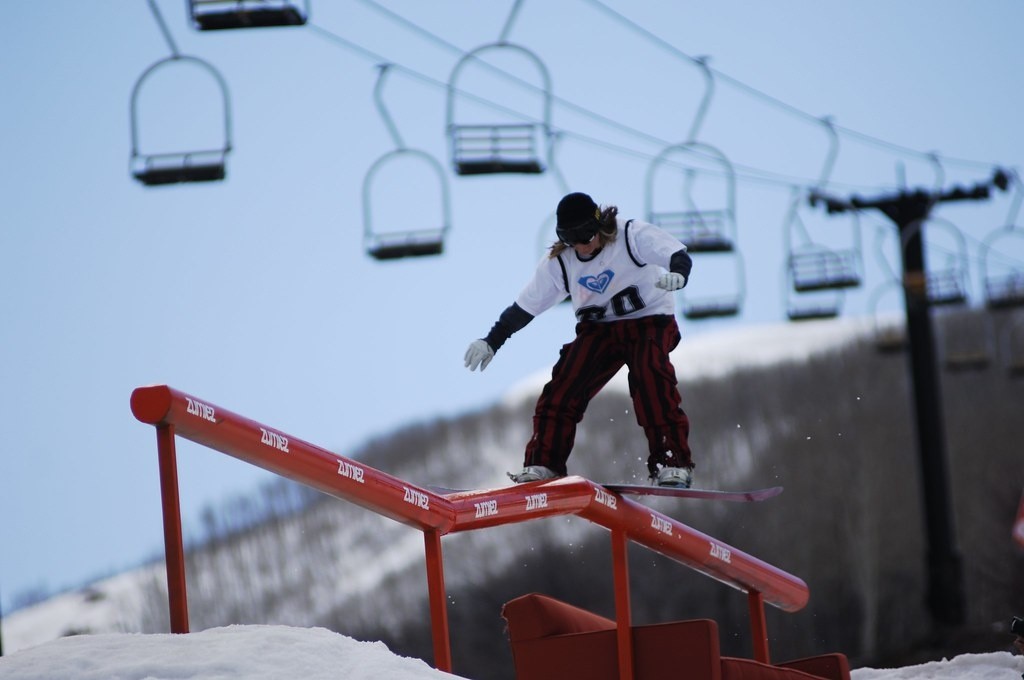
[515,466,555,482]
[658,467,694,489]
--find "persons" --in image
[463,193,693,490]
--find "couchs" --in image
[501,592,852,680]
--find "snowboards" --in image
[426,482,785,505]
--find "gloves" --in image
[463,339,494,373]
[655,272,685,292]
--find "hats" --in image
[556,192,602,242]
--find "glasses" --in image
[562,236,595,247]
